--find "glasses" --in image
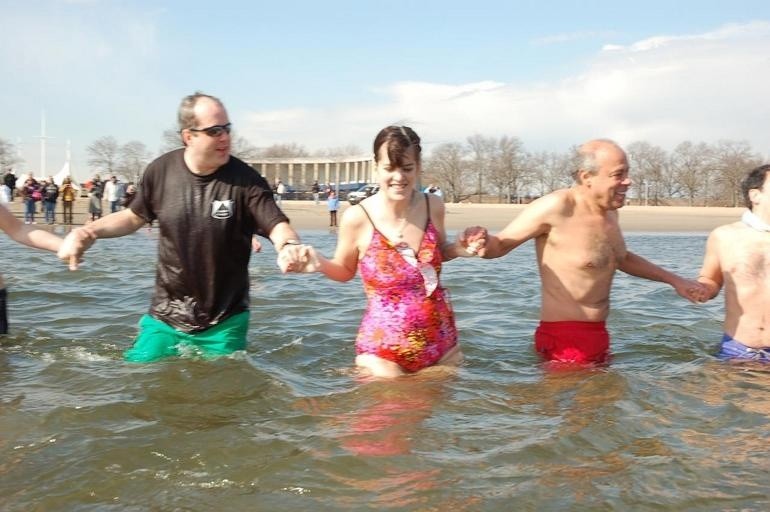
[185,123,231,137]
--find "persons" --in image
[102,176,125,213]
[86,174,105,222]
[463,139,713,370]
[328,191,340,226]
[121,182,137,208]
[273,177,286,207]
[687,164,770,363]
[276,125,489,380]
[0,200,95,335]
[325,182,334,198]
[20,172,43,224]
[58,176,79,223]
[311,180,323,203]
[64,91,308,366]
[39,176,59,222]
[3,168,19,202]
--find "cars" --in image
[348,185,379,204]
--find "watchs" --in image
[278,239,300,251]
